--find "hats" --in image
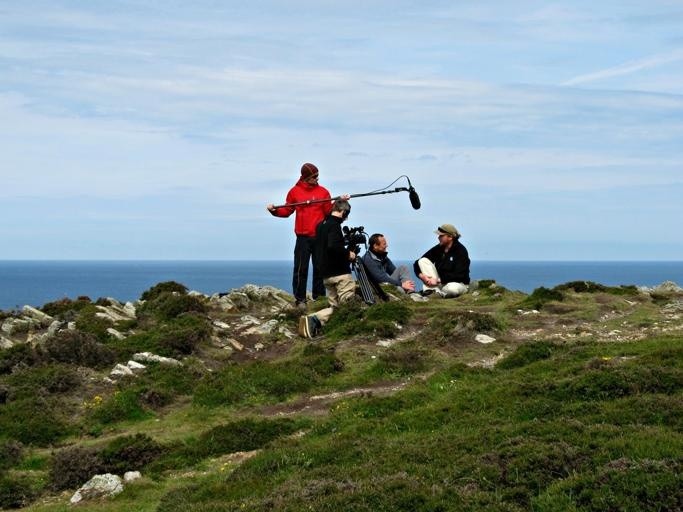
[301,162,320,180]
[432,223,459,238]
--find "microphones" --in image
[407,177,420,209]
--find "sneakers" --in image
[418,289,434,297]
[296,313,316,339]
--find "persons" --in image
[297,199,356,341]
[361,234,429,302]
[412,224,471,298]
[267,163,351,308]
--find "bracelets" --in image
[436,278,440,283]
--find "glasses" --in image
[305,173,320,179]
[436,225,453,235]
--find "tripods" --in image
[350,249,390,306]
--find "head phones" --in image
[342,203,350,218]
